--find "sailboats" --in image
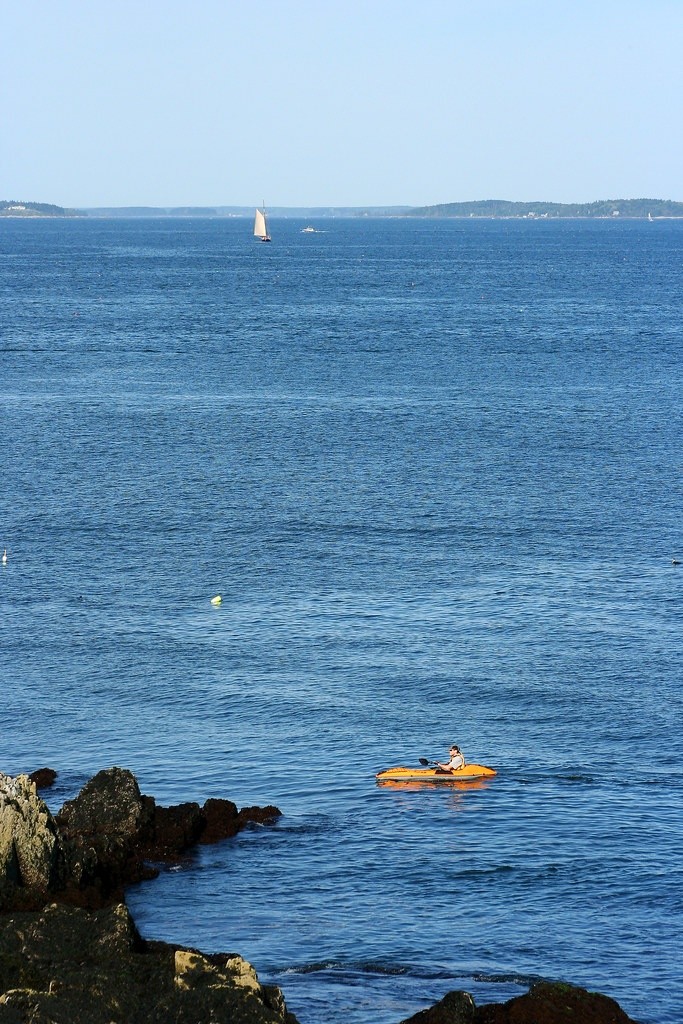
[252,208,272,244]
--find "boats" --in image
[375,763,497,787]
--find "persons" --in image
[433,746,465,774]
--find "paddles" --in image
[418,757,439,766]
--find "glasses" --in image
[450,749,452,751]
